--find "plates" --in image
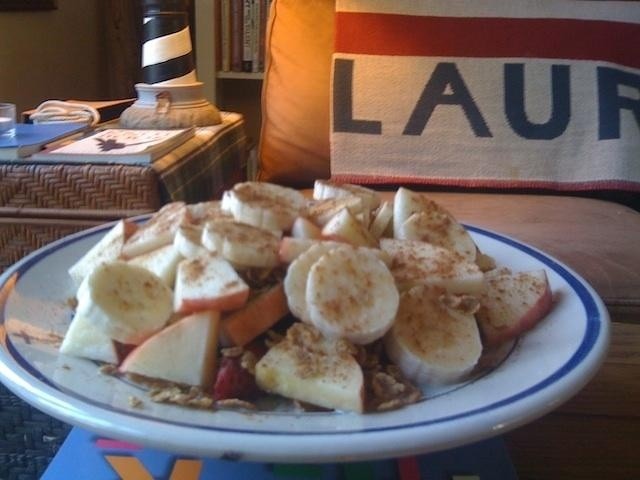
[0,213,615,464]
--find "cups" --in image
[0,103,17,137]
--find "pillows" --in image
[256,0,640,188]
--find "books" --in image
[33,127,196,163]
[218,0,269,74]
[0,123,87,161]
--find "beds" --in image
[256,180,640,317]
